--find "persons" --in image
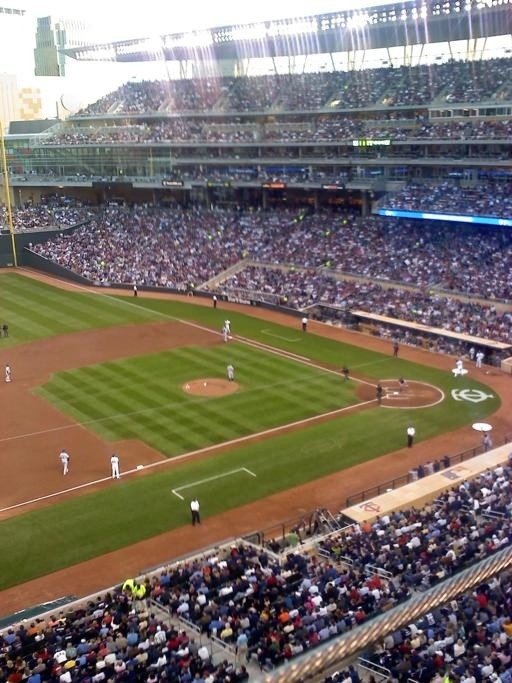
[407,422,415,447]
[376,381,383,406]
[396,375,407,395]
[58,448,70,477]
[453,357,464,377]
[0,453,511,683]
[1,50,512,365]
[340,364,350,381]
[475,347,485,369]
[4,362,12,383]
[226,362,235,382]
[482,429,492,451]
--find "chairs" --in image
[0,474,511,683]
